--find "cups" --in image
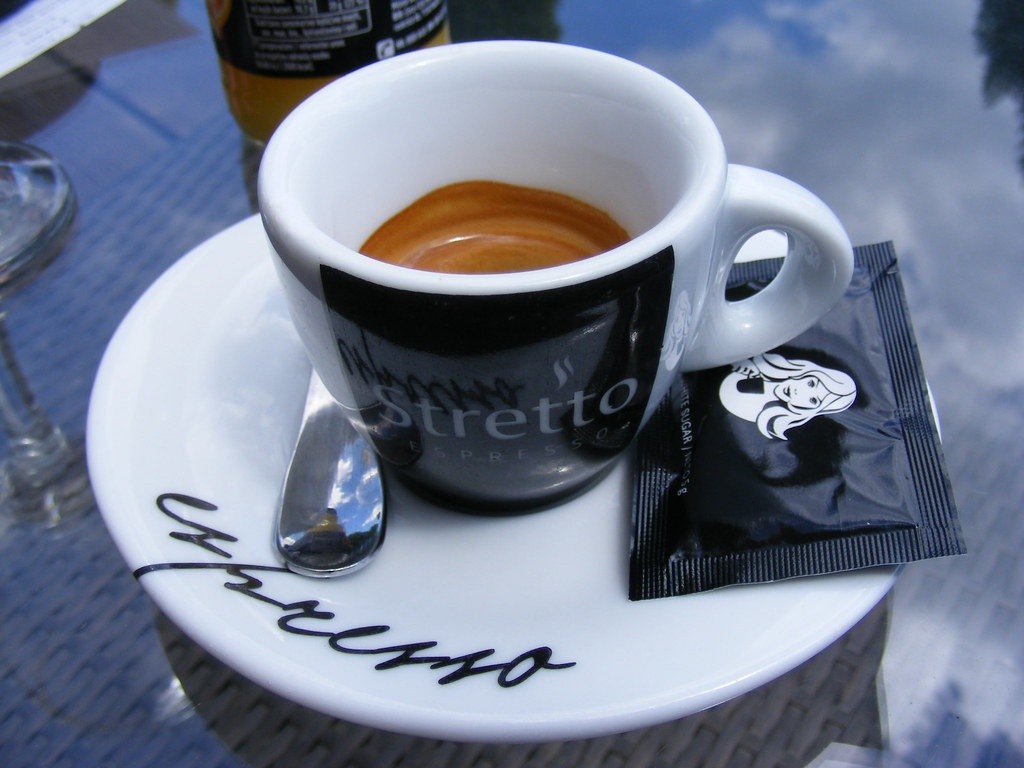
[258,41,855,514]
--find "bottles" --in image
[207,1,453,150]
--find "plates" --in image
[88,213,939,744]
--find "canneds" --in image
[205,0,452,149]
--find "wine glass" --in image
[0,143,232,731]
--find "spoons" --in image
[271,367,389,578]
